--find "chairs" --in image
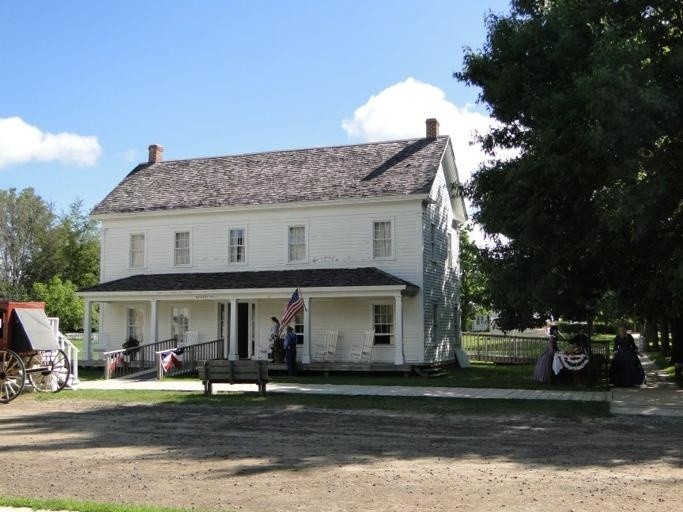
[348,329,375,363]
[312,327,339,363]
[178,330,199,360]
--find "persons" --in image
[534,326,559,384]
[568,329,587,347]
[271,317,280,363]
[609,327,646,386]
[283,327,298,374]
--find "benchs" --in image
[195,358,271,398]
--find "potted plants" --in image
[120,337,139,360]
[271,334,286,363]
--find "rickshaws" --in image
[0,301,71,403]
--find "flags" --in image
[279,288,304,336]
